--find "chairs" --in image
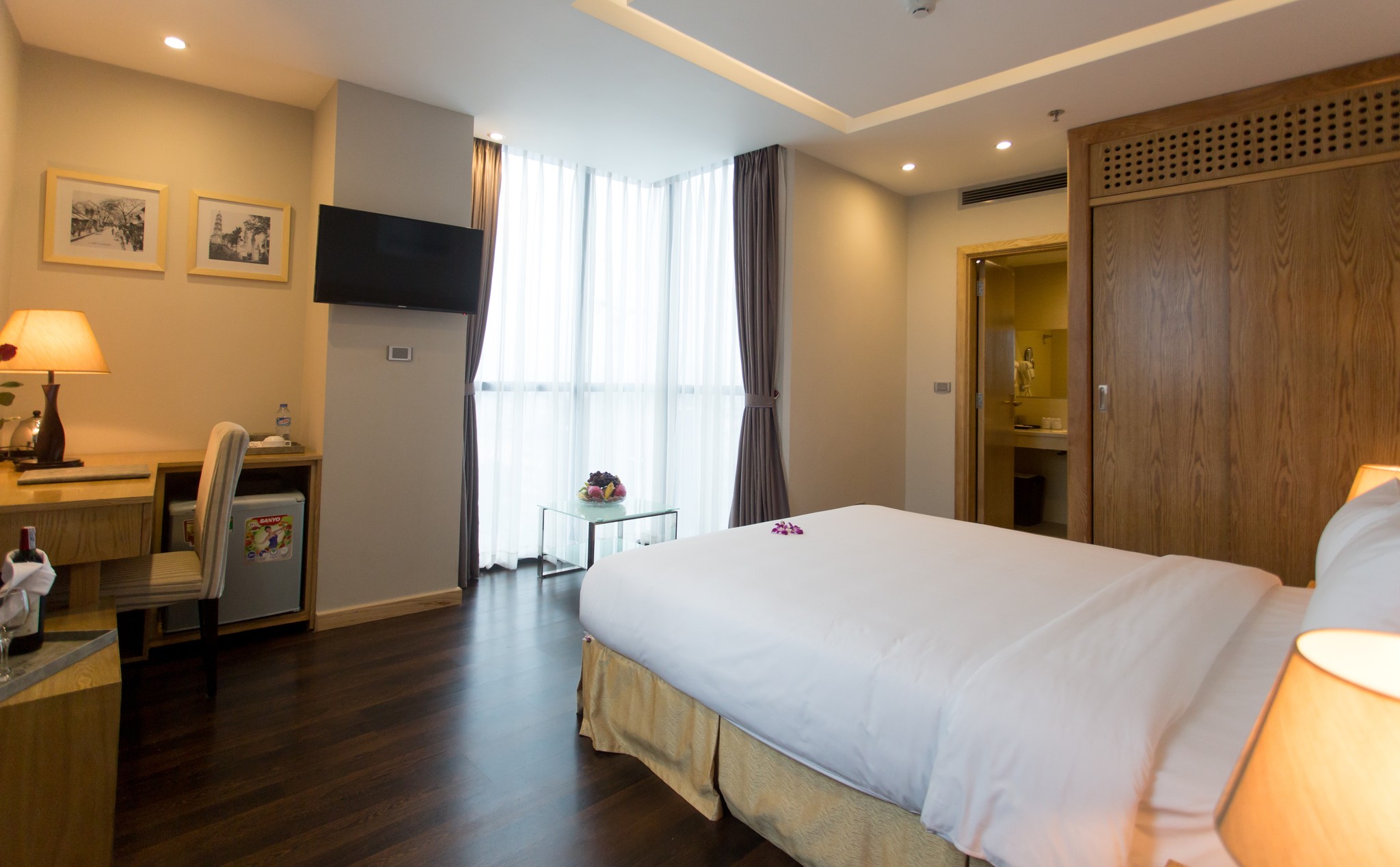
[41,421,249,716]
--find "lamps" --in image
[1345,464,1400,501]
[0,309,112,470]
[1212,627,1400,867]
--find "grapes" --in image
[587,471,619,487]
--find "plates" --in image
[577,492,625,507]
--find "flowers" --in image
[772,521,803,535]
[0,343,24,430]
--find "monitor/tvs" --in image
[312,204,484,317]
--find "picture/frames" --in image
[42,167,167,273]
[186,189,291,282]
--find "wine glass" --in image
[0,588,31,677]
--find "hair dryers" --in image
[1023,361,1033,383]
[1014,360,1019,380]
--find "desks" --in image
[0,442,323,661]
[537,501,680,579]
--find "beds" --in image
[576,506,1316,867]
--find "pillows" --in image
[1316,477,1400,582]
[1297,513,1400,632]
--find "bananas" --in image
[604,482,614,499]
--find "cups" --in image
[1041,419,1052,429]
[262,436,291,447]
[1051,419,1062,429]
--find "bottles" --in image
[275,404,292,440]
[9,526,45,657]
[0,626,10,683]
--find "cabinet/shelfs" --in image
[1091,158,1400,587]
[0,603,121,867]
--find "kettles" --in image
[9,410,43,456]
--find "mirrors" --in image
[1014,329,1069,399]
